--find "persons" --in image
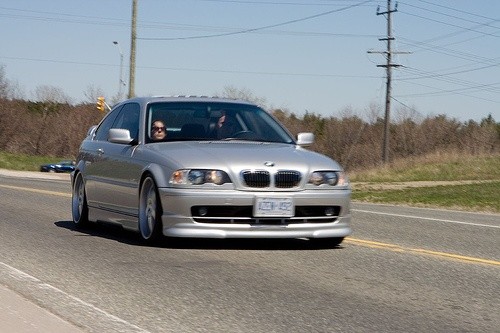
[151,119,169,142]
[215,113,239,140]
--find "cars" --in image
[40,160,76,173]
[70,96,352,244]
